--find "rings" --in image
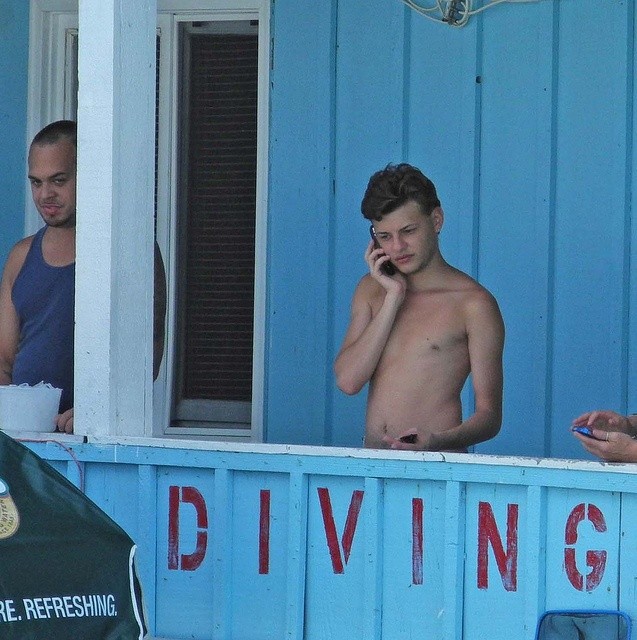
[606,431,609,440]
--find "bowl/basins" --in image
[0,387,63,433]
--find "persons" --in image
[0,120,167,433]
[569,409,637,464]
[333,162,505,453]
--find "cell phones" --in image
[571,426,596,438]
[370,225,395,275]
